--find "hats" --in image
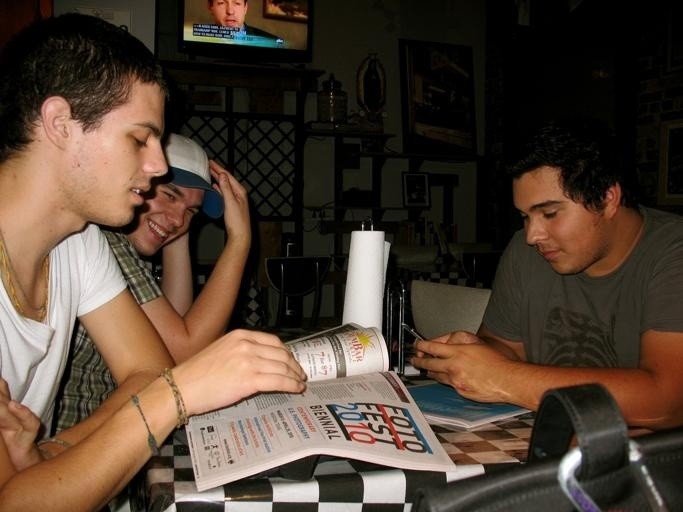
[161,132,226,220]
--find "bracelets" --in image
[36,438,74,450]
[130,395,159,456]
[157,369,188,428]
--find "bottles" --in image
[316,72,347,122]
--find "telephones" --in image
[344,187,375,210]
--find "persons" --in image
[411,110,683,433]
[208,1,277,38]
[51,133,253,436]
[0,13,307,511]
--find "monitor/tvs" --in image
[154,0,313,80]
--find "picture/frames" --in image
[652,111,682,213]
[396,37,478,162]
[399,170,431,211]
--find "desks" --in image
[125,362,654,512]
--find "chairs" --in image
[265,254,334,332]
[407,278,493,342]
[459,249,503,291]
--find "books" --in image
[186,323,457,494]
[406,383,534,434]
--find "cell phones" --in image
[402,322,426,340]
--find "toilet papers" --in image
[342,230,391,334]
